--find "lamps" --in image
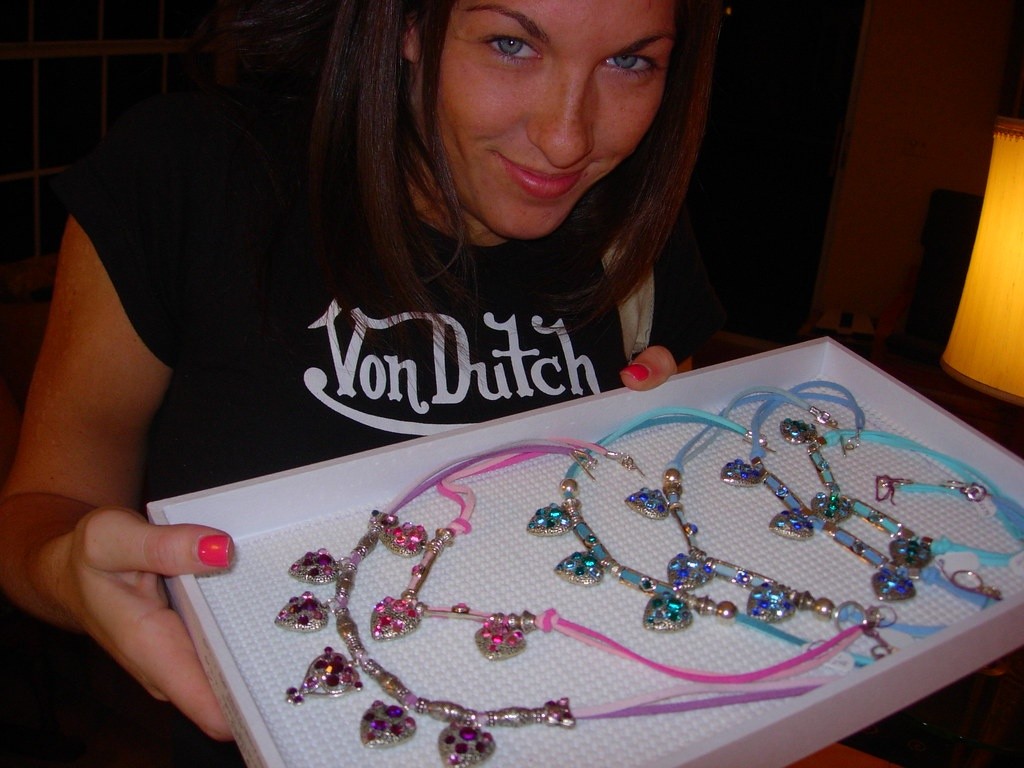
[939,118,1024,407]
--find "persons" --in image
[0,0,731,743]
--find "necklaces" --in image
[273,381,1024,768]
